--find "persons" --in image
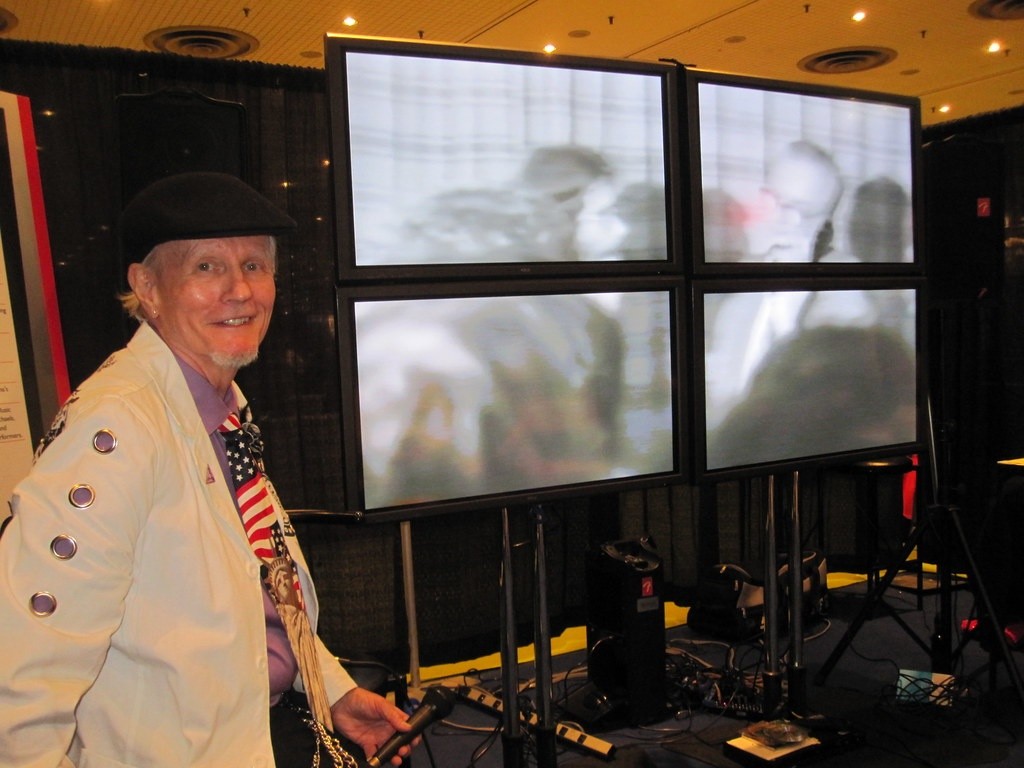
[0,169,423,768]
[376,132,915,508]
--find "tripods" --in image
[815,295,1024,706]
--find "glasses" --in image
[580,203,629,223]
[753,189,830,227]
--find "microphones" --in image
[368,684,455,768]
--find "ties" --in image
[217,408,335,734]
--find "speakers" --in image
[920,133,1007,298]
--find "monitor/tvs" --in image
[320,32,929,525]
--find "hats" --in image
[116,172,301,265]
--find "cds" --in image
[746,721,808,748]
